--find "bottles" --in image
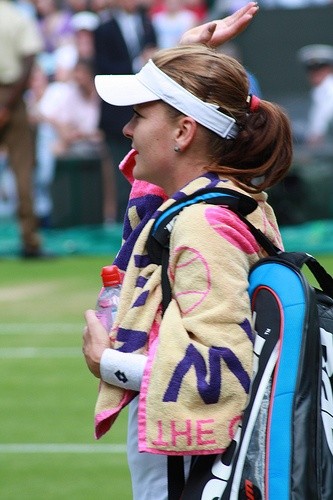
[95,265,123,333]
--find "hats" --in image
[94,58,242,140]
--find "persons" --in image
[83,2,294,500]
[0,0,205,261]
[299,45,333,145]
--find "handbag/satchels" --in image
[149,188,333,499]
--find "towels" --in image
[93,148,283,459]
